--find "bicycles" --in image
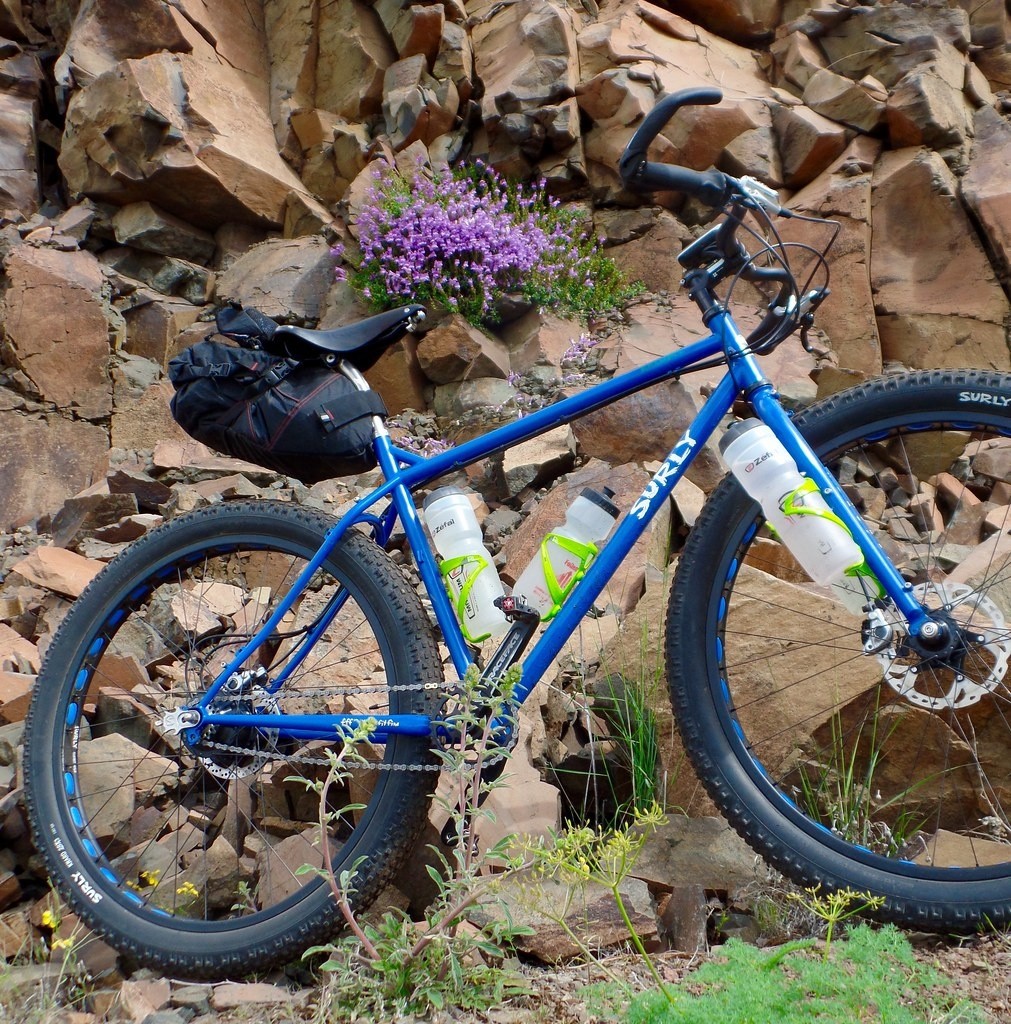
[23,87,1011,980]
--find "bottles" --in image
[511,486,621,618]
[424,485,512,639]
[832,569,880,617]
[717,416,864,585]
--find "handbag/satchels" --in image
[166,302,390,484]
